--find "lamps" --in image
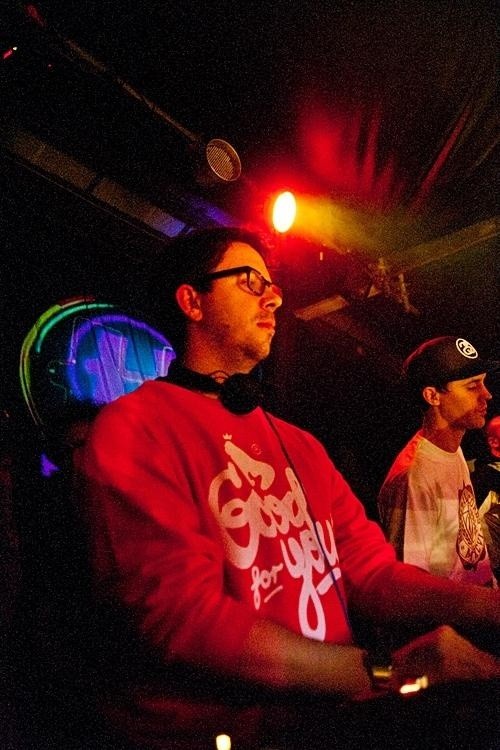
[189,136,298,238]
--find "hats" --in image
[402,337,498,395]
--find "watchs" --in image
[361,646,394,695]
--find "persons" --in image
[464,409,500,519]
[69,221,500,749]
[4,389,105,707]
[373,333,500,596]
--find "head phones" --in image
[165,360,271,416]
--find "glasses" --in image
[205,266,282,301]
